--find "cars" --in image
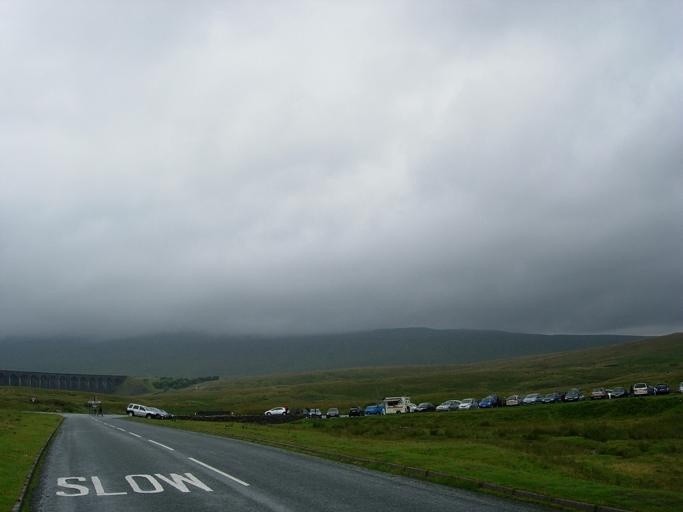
[125,402,170,421]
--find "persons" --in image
[303,407,310,423]
[32,396,39,408]
[92,403,104,416]
